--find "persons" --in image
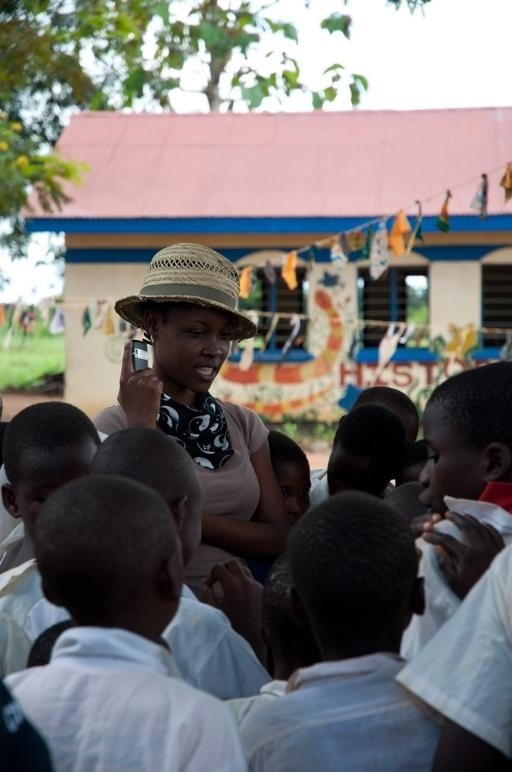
[23,429,273,699]
[0,401,100,625]
[0,475,248,772]
[228,492,439,771]
[88,239,290,602]
[268,362,510,772]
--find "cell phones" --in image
[132,337,148,374]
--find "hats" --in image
[115,241,258,342]
